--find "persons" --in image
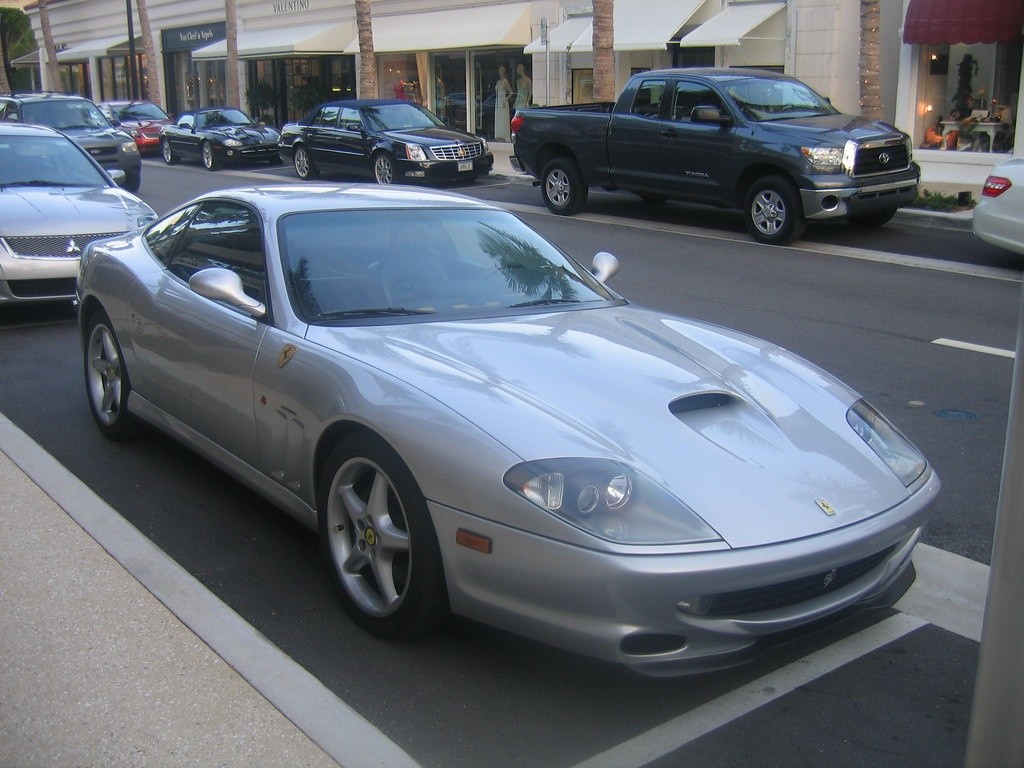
[495,66,513,143]
[394,70,407,100]
[514,64,531,109]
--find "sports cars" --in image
[159,108,283,171]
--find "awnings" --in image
[524,0,786,54]
[903,0,1024,44]
[11,33,145,67]
[191,2,532,62]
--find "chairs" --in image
[0,148,46,181]
[286,217,457,315]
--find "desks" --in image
[940,121,1004,153]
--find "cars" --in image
[973,157,1024,255]
[90,101,177,157]
[69,182,944,669]
[0,122,159,305]
[277,99,494,186]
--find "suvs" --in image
[0,89,141,191]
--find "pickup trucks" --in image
[508,67,921,247]
